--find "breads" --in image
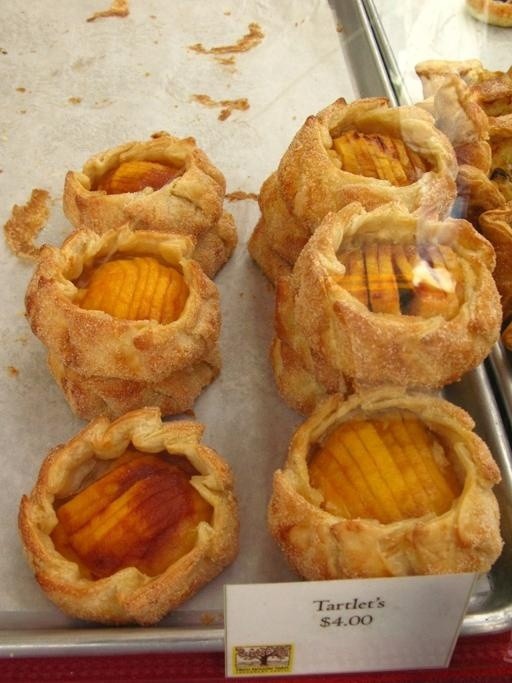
[246,0,512,626]
[18,130,242,630]
